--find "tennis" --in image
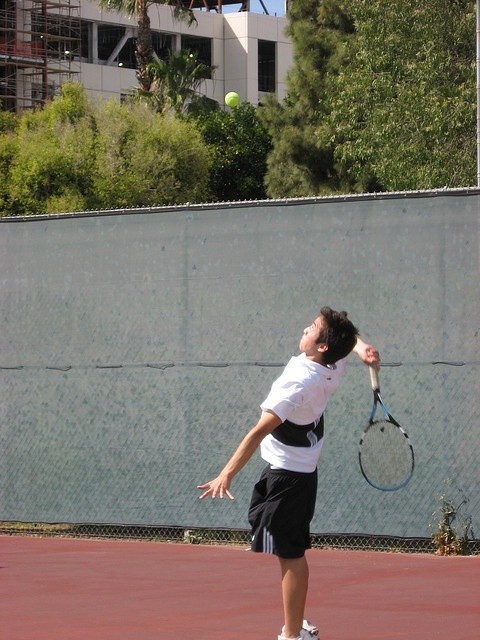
[225,92,239,107]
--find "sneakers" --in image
[278,628,319,640]
[281,619,319,636]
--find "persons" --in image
[196,306,380,640]
